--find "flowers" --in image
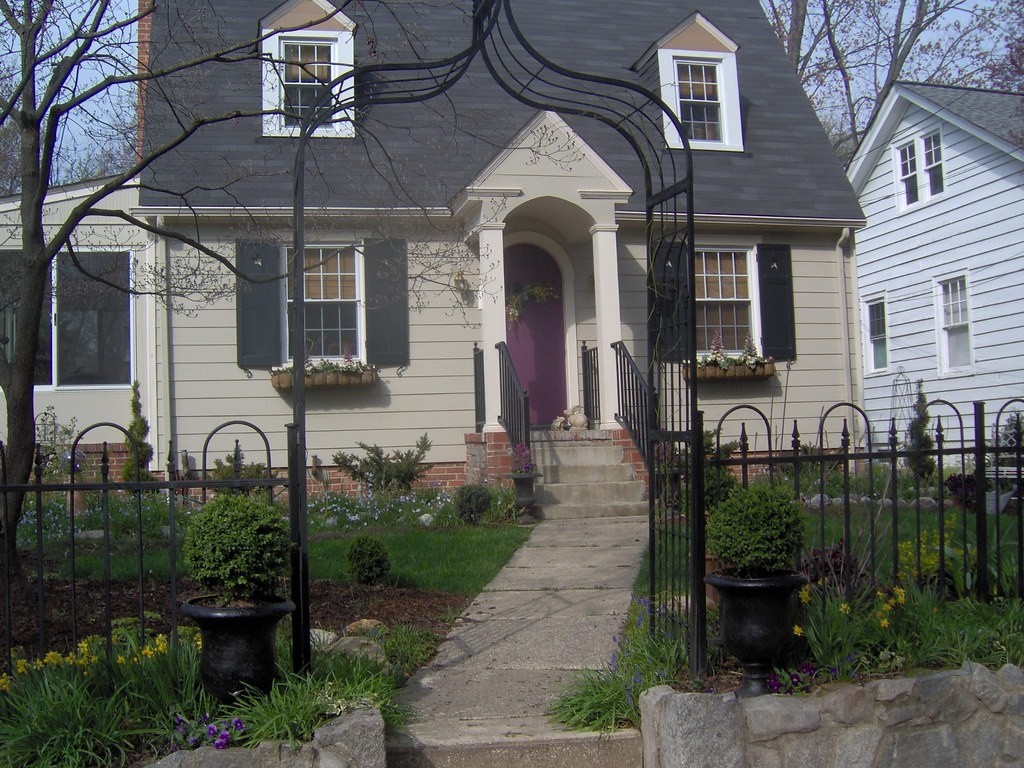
[652,427,680,466]
[510,443,537,473]
[506,281,561,330]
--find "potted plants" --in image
[679,328,775,383]
[178,481,300,715]
[693,429,760,611]
[707,480,827,693]
[268,345,380,392]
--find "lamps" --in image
[454,269,468,293]
[60,448,87,475]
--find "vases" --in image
[657,467,685,509]
[505,470,546,525]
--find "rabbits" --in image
[563,405,589,431]
[551,416,566,431]
